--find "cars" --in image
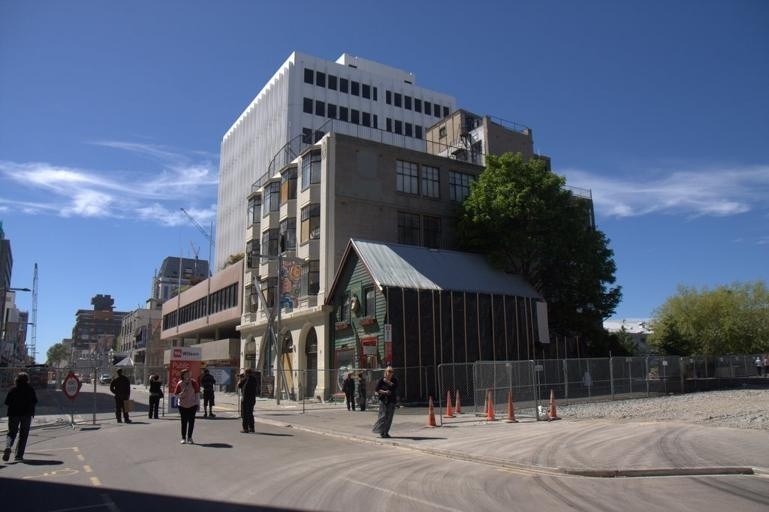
[70,371,112,386]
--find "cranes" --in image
[190,240,200,260]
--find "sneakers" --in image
[124,420,132,423]
[208,412,216,417]
[117,420,122,423]
[240,429,255,433]
[187,438,193,444]
[180,439,187,444]
[2,447,24,464]
[204,413,208,417]
[380,433,391,438]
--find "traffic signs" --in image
[206,367,232,385]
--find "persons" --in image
[238,368,258,434]
[235,373,246,393]
[2,370,39,463]
[146,374,164,420]
[356,373,367,412]
[201,369,217,417]
[754,356,762,377]
[342,373,356,412]
[109,368,134,424]
[374,366,400,439]
[763,356,769,378]
[173,368,201,446]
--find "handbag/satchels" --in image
[159,389,163,398]
[374,390,385,400]
[123,399,136,413]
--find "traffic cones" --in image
[478,389,489,414]
[545,388,563,420]
[423,395,440,429]
[480,391,499,422]
[451,389,462,415]
[441,391,457,418]
[500,390,520,424]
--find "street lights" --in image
[3,322,37,380]
[0,288,32,334]
[248,251,308,406]
